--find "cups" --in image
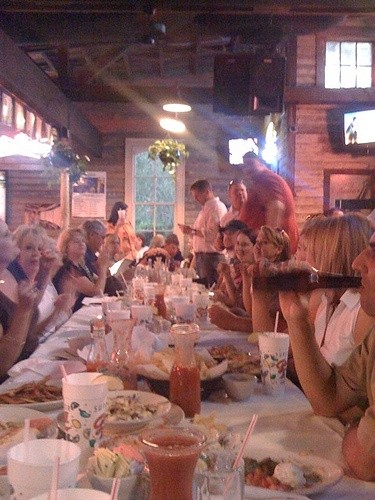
[31,487,110,500]
[118,209,125,220]
[103,273,209,341]
[258,331,290,396]
[7,438,81,500]
[85,467,139,500]
[194,448,246,500]
[137,423,207,500]
[61,373,109,467]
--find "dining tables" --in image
[0,244,375,500]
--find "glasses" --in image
[276,226,284,237]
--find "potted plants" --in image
[41,143,90,184]
[151,140,187,173]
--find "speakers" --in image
[213,53,286,115]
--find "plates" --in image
[243,450,344,495]
[0,383,186,467]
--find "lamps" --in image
[163,90,192,112]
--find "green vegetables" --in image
[244,457,278,475]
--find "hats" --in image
[220,220,247,233]
[227,179,244,190]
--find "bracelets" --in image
[192,229,196,234]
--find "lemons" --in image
[248,332,268,343]
[196,456,208,470]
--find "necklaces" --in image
[0,280,4,284]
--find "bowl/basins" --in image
[146,378,220,401]
[222,374,257,401]
[103,390,170,436]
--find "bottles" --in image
[87,320,109,375]
[109,319,137,390]
[252,266,362,292]
[170,324,202,417]
[134,255,171,284]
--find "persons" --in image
[182,150,345,358]
[13,222,75,340]
[83,220,106,275]
[288,214,375,391]
[52,228,113,313]
[0,218,56,376]
[101,201,183,267]
[271,208,375,480]
